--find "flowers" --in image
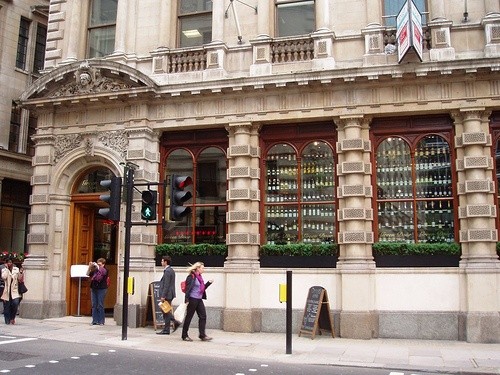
[0,249,28,261]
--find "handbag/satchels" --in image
[18,281,28,294]
[158,300,172,313]
[181,274,194,293]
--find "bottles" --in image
[265,143,335,244]
[378,136,455,244]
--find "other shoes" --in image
[91,322,104,325]
[156,330,170,335]
[201,335,213,341]
[173,322,182,332]
[184,337,193,341]
[11,319,15,324]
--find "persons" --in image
[86,258,109,327]
[1,258,29,325]
[181,262,214,341]
[80,73,91,88]
[155,256,181,335]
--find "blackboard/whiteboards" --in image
[300,286,334,333]
[144,281,174,325]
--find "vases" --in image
[0,259,23,263]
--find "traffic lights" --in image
[98,177,122,222]
[141,190,157,220]
[169,173,193,221]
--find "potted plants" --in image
[155,243,225,267]
[260,243,336,268]
[371,241,461,267]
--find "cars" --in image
[0,263,22,302]
